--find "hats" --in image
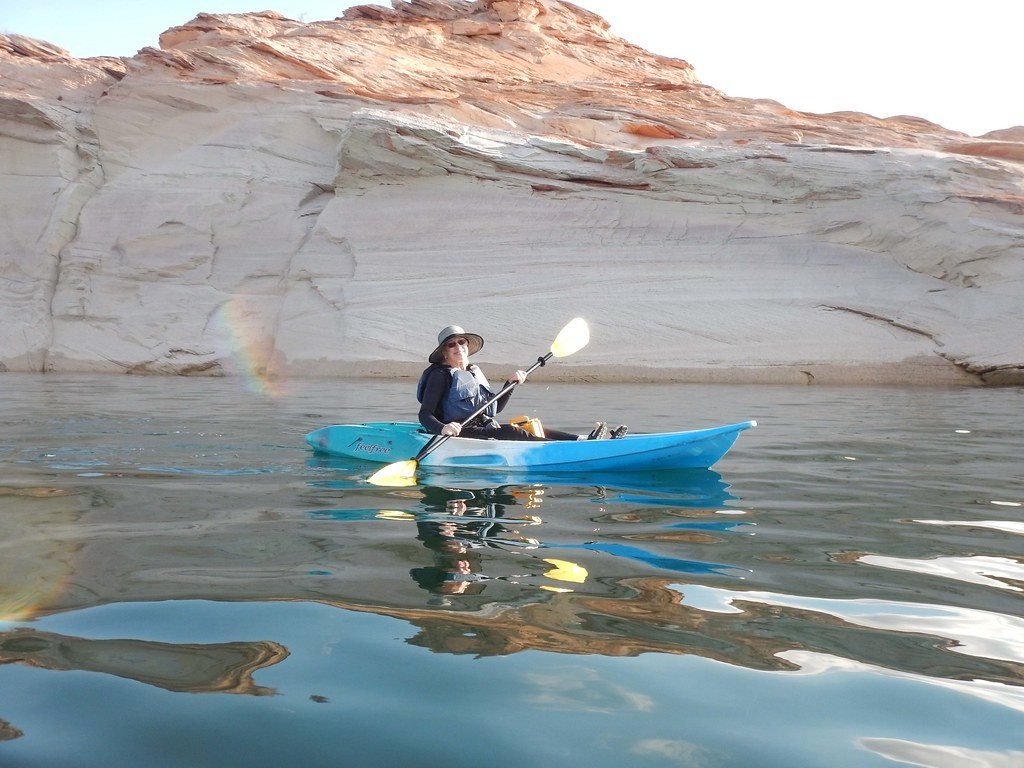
[428,325,484,363]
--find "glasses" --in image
[445,339,468,348]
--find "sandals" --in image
[609,425,628,439]
[587,421,607,440]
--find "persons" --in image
[419,325,628,440]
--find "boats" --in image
[303,422,758,469]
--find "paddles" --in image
[365,316,591,486]
